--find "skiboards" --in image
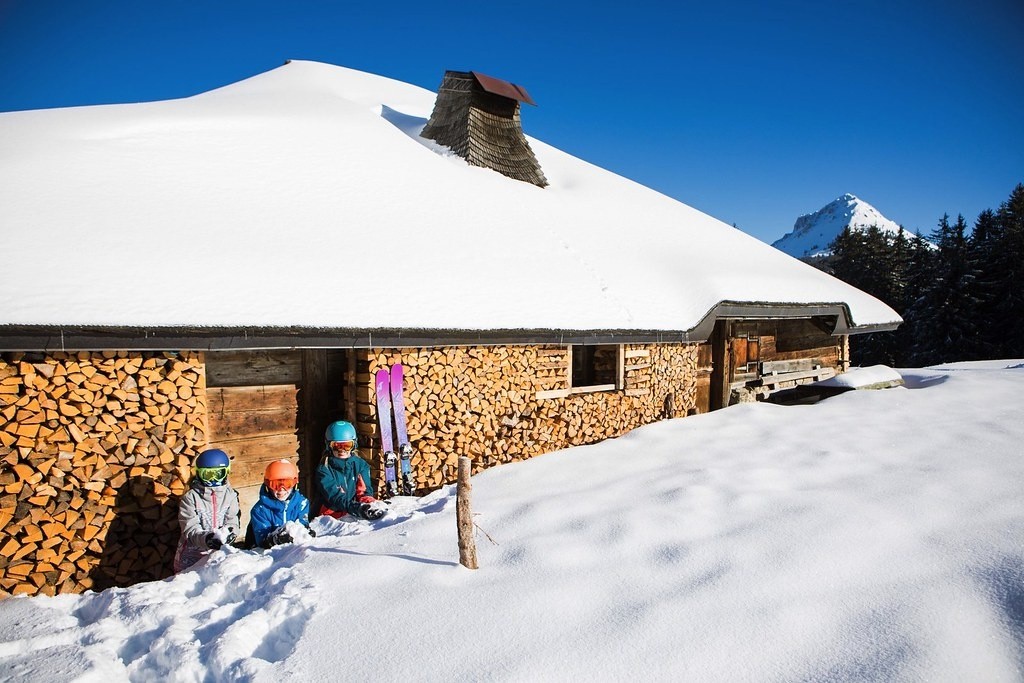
[374,363,416,498]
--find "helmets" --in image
[197,450,229,487]
[265,460,297,480]
[324,420,358,450]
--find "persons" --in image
[174,449,242,576]
[250,458,310,552]
[311,421,389,521]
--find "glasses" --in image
[265,480,297,491]
[198,467,229,481]
[328,441,354,451]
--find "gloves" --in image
[352,501,391,519]
[206,532,222,550]
[262,528,293,549]
[231,541,252,550]
[304,524,316,537]
